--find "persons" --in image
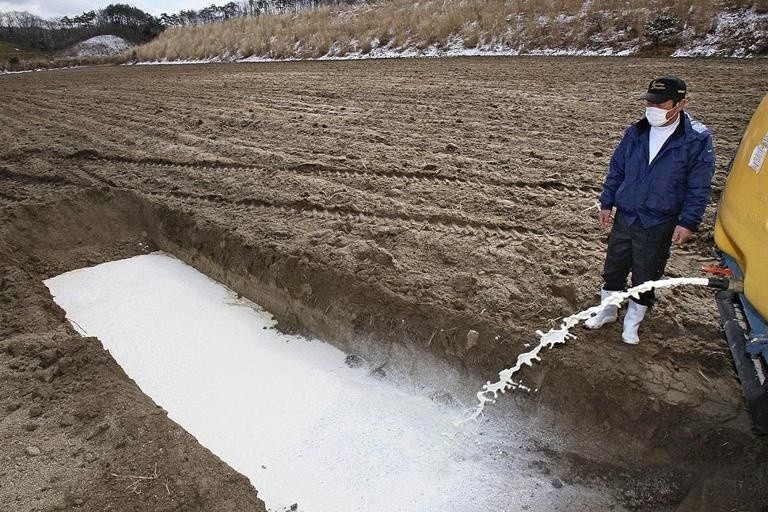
[584,77,716,346]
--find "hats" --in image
[640,76,686,103]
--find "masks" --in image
[645,102,679,127]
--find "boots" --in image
[583,288,623,330]
[622,298,648,345]
